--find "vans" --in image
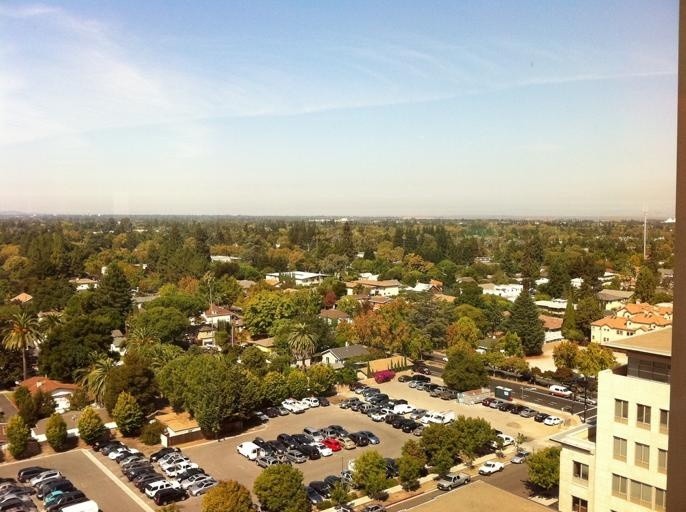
[548,384,573,398]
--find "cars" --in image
[436,471,471,491]
[482,397,564,427]
[338,385,456,436]
[0,465,98,512]
[92,439,219,506]
[304,457,400,512]
[485,430,515,450]
[253,396,330,423]
[510,451,531,464]
[575,394,597,405]
[478,460,505,476]
[236,424,380,469]
[398,374,459,400]
